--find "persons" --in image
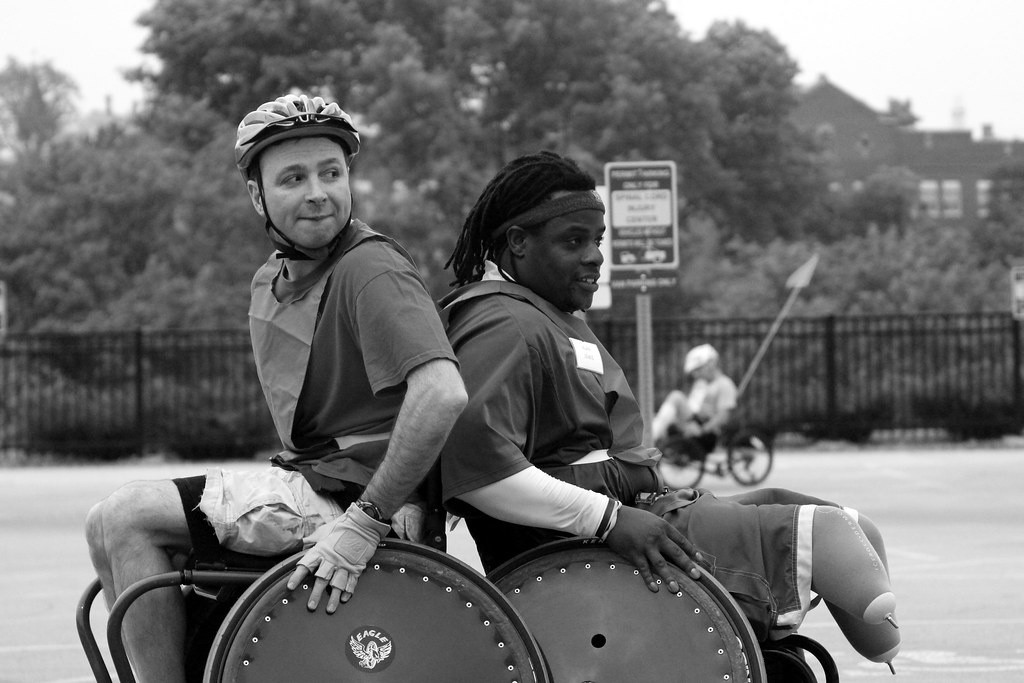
[652,343,737,447]
[436,149,901,665]
[85,95,469,683]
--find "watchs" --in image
[356,498,392,525]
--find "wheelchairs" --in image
[77,524,839,683]
[652,395,774,494]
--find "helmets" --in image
[681,344,723,375]
[234,94,361,183]
